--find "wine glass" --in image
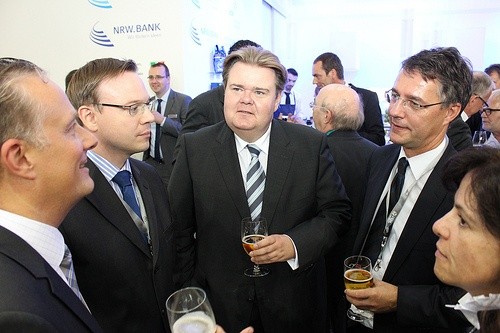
[343,256,373,322]
[240,217,270,277]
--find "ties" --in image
[358,157,409,268]
[244,143,265,227]
[155,99,163,164]
[61,244,79,299]
[284,92,290,105]
[115,171,148,242]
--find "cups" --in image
[165,287,217,333]
[472,131,487,147]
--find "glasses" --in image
[384,88,455,113]
[473,92,489,108]
[86,99,154,117]
[479,106,500,115]
[310,102,328,110]
[147,75,168,81]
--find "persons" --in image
[0,57,253,333]
[166,46,351,333]
[433,146,500,333]
[142,40,500,187]
[341,47,472,333]
[58,58,176,333]
